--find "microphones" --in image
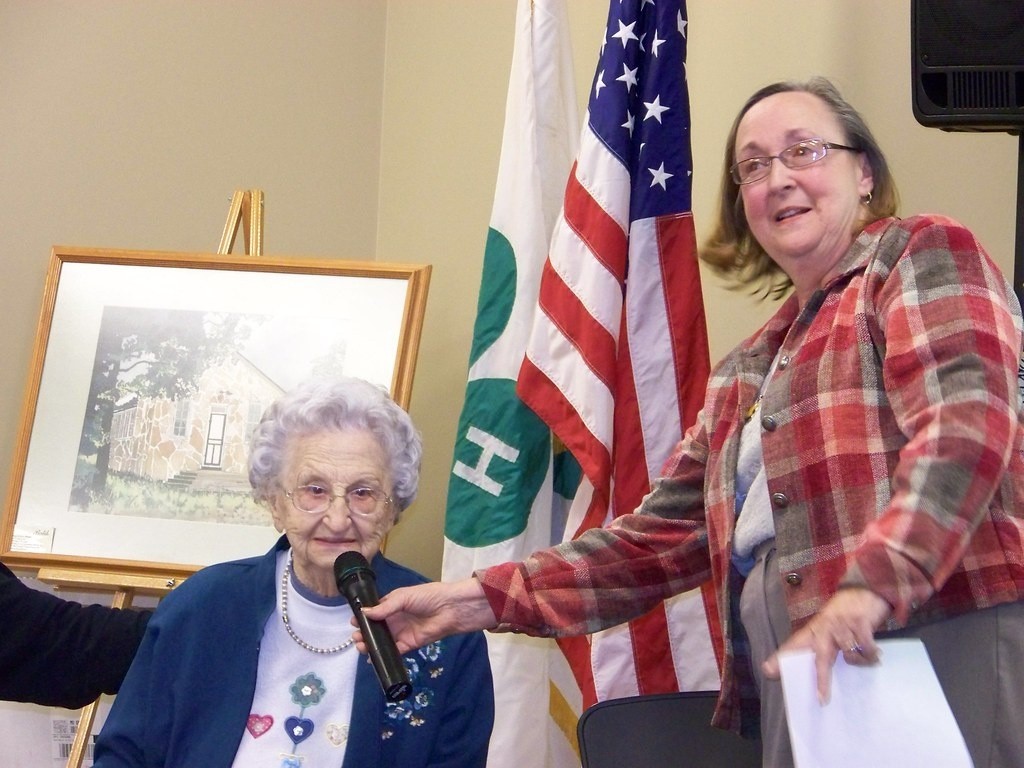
[333,550,414,701]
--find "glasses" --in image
[730,138,860,186]
[274,484,395,520]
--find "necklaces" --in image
[281,556,360,656]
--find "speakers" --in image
[911,0,1024,133]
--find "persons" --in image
[0,563,154,709]
[351,77,1023,768]
[93,377,495,768]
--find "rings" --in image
[842,644,863,654]
